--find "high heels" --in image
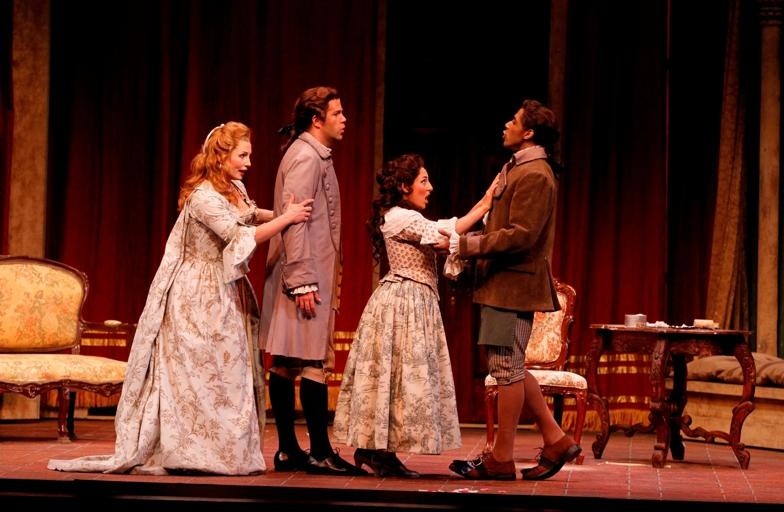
[353,446,376,470]
[371,451,423,480]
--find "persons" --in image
[431,100,582,482]
[333,154,501,477]
[45,120,315,475]
[259,88,367,476]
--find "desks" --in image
[589,325,756,469]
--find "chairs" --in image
[485,278,588,465]
[0,254,129,444]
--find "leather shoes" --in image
[307,450,369,477]
[274,451,308,472]
[449,451,517,482]
[521,434,582,479]
